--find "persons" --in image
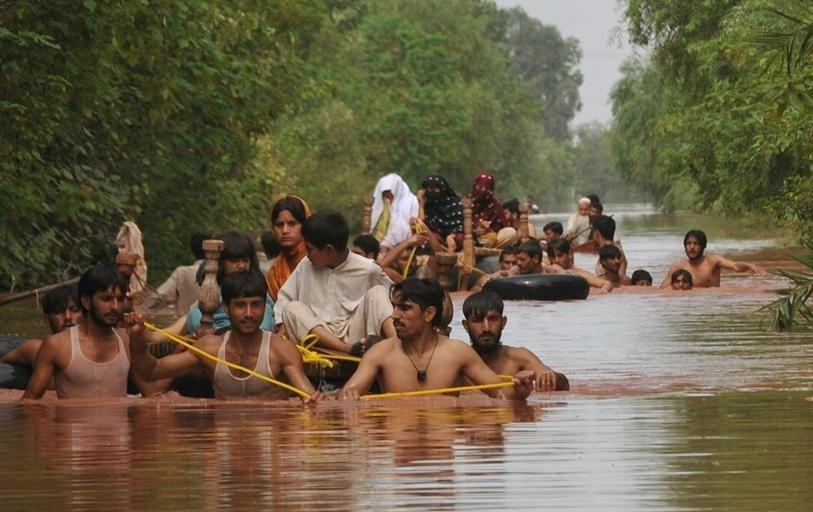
[262,192,313,303]
[258,230,281,274]
[123,228,276,348]
[659,228,762,290]
[0,282,84,391]
[18,263,218,403]
[369,170,654,297]
[350,234,380,260]
[272,207,399,358]
[140,231,217,320]
[460,289,570,394]
[669,269,693,292]
[122,267,332,404]
[334,276,538,404]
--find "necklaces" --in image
[401,332,439,380]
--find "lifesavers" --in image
[1,335,33,389]
[483,274,589,299]
